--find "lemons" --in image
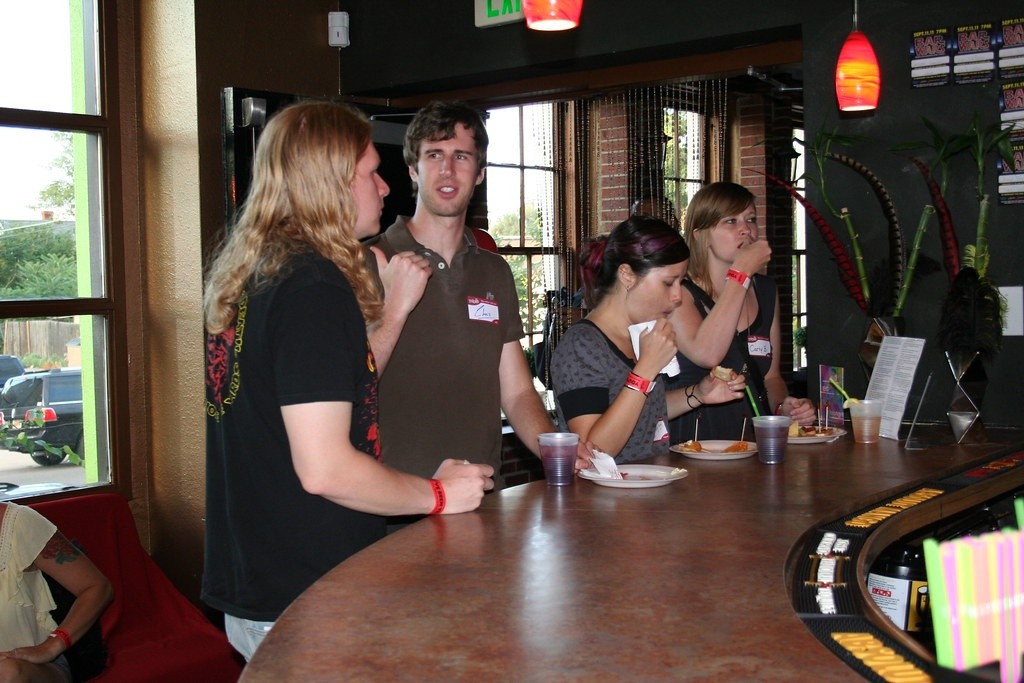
[843,398,861,409]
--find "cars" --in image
[0,367,87,466]
[0,353,28,390]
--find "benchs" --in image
[30,493,248,683]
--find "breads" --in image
[713,365,734,382]
[789,420,799,437]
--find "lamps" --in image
[835,0,881,111]
[522,0,583,31]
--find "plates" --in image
[577,464,688,488]
[787,426,847,444]
[669,440,758,460]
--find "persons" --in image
[203,99,496,664]
[550,215,746,463]
[667,181,817,443]
[631,195,672,220]
[359,101,603,534]
[0,501,114,683]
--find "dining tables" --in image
[237,422,1024,683]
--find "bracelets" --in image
[726,268,751,290]
[775,402,782,416]
[429,479,446,514]
[684,384,702,409]
[52,627,73,650]
[623,372,656,398]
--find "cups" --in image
[850,400,882,443]
[538,432,579,485]
[752,416,791,463]
